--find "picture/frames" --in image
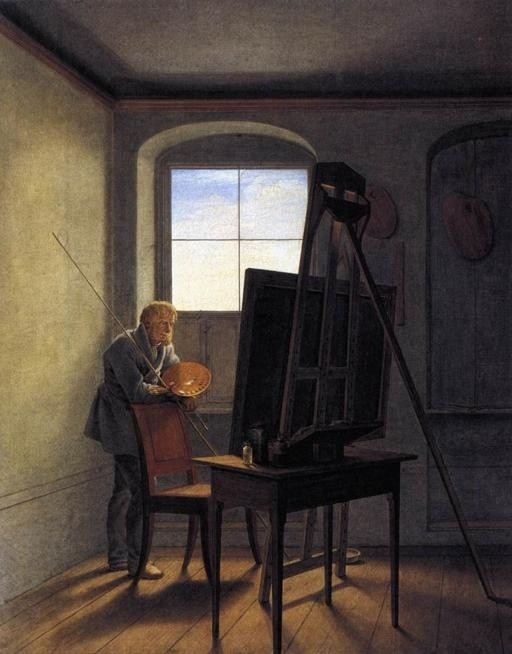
[229,268,397,455]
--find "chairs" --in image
[126,401,263,585]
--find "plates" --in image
[159,362,212,396]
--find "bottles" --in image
[241,423,289,472]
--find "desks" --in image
[190,446,420,654]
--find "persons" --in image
[84,300,200,581]
